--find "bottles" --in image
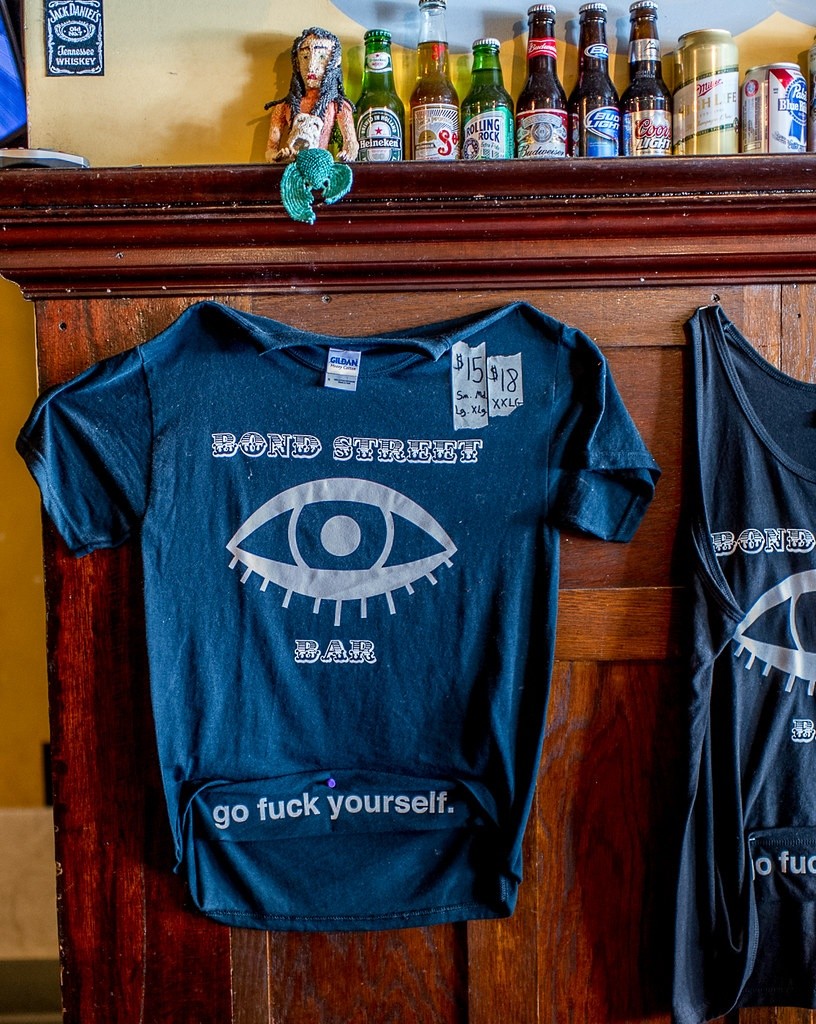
[566,2,621,158]
[512,2,571,158]
[619,0,673,158]
[408,0,460,162]
[459,36,517,162]
[355,29,405,163]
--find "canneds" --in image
[808,34,816,151]
[673,28,740,153]
[740,61,808,153]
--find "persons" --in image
[264,25,359,166]
